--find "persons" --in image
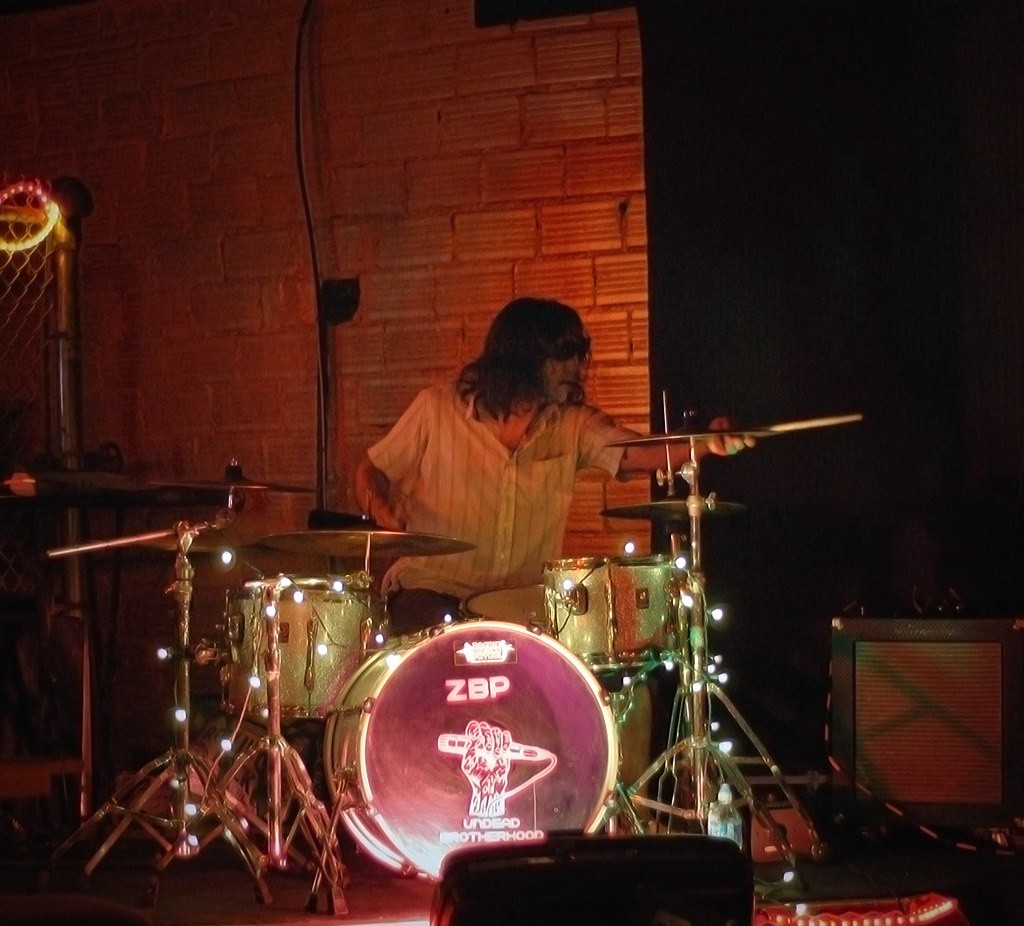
[354,295,759,637]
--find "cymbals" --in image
[603,423,778,448]
[145,475,315,494]
[257,520,476,558]
[600,493,746,521]
[139,533,276,554]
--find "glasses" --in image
[544,338,585,361]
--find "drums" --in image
[220,571,378,721]
[323,618,624,887]
[455,581,651,833]
[540,551,679,665]
